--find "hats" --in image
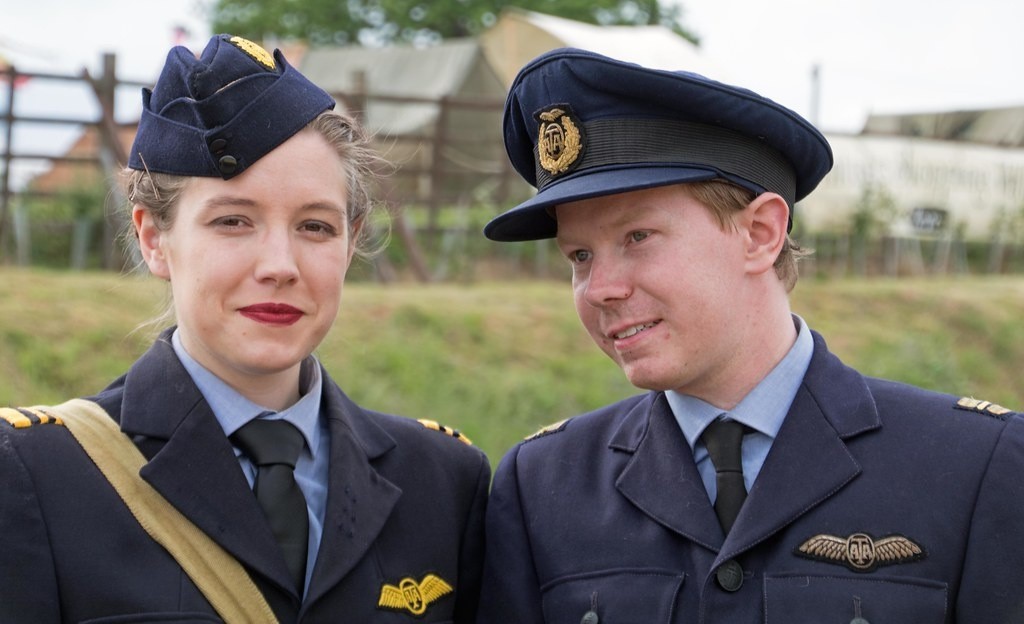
[484,48,834,241]
[127,34,337,181]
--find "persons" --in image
[481,47,1024,624]
[0,34,490,624]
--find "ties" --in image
[696,417,760,545]
[228,419,308,598]
